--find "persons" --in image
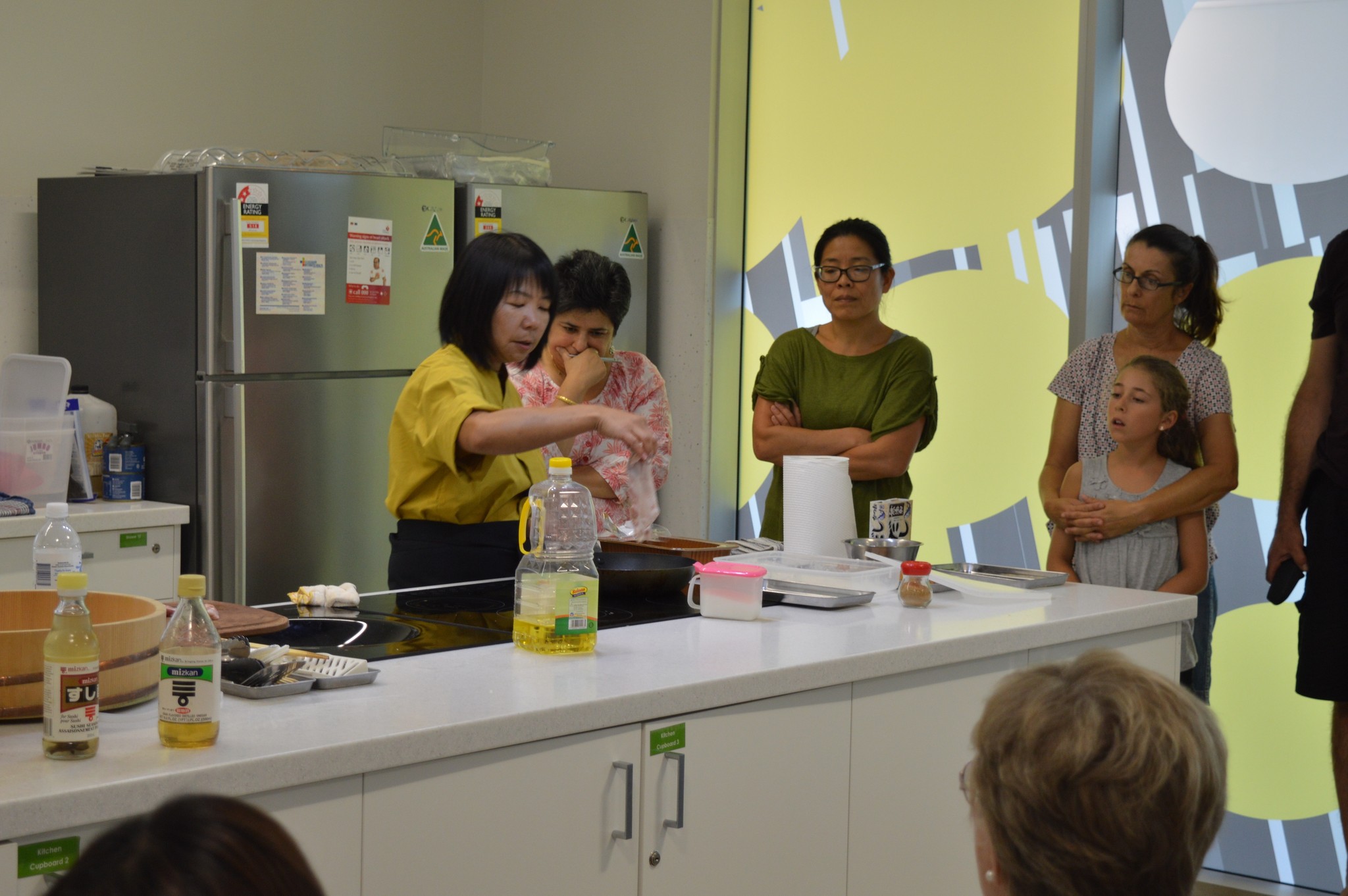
[504,250,672,553]
[386,231,658,590]
[957,648,1228,896]
[1045,355,1208,688]
[1038,224,1239,705]
[1267,232,1347,852]
[44,792,326,896]
[751,217,939,540]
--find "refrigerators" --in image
[37,168,651,607]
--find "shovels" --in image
[241,660,306,687]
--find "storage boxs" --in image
[0,353,76,507]
[381,124,555,180]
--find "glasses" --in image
[1113,267,1185,290]
[817,263,885,282]
[960,761,976,805]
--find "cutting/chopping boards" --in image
[163,600,289,635]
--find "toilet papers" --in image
[781,453,856,559]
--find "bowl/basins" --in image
[842,538,924,562]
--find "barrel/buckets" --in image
[513,457,599,656]
[102,440,145,502]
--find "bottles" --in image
[42,572,99,761]
[66,385,116,497]
[159,574,222,749]
[898,561,932,609]
[32,502,83,590]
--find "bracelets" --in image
[554,395,577,406]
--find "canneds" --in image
[102,470,146,502]
[102,432,146,474]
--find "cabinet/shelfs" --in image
[0,623,1186,896]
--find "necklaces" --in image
[551,354,565,376]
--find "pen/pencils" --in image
[568,354,624,363]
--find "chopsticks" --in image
[222,639,330,659]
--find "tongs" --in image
[220,636,251,659]
